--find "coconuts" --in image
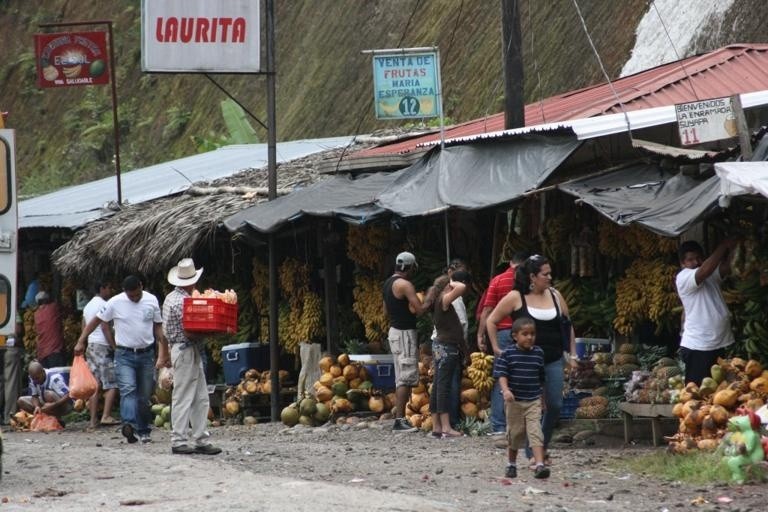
[401,360,499,434]
[667,356,768,453]
[222,368,279,425]
[150,377,213,430]
[281,353,398,426]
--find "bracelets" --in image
[567,355,578,359]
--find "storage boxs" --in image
[347,351,396,390]
[219,341,268,388]
[559,388,593,420]
[571,336,613,363]
[46,366,71,385]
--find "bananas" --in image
[553,276,611,337]
[352,276,388,344]
[144,276,171,298]
[407,220,490,339]
[188,272,256,364]
[729,238,757,278]
[347,229,387,270]
[611,225,689,335]
[23,309,36,360]
[251,277,271,344]
[467,352,495,390]
[730,297,768,355]
[59,288,84,349]
[277,292,321,353]
[279,257,311,297]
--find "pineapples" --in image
[575,342,674,420]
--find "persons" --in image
[161,258,223,455]
[32,290,70,369]
[2,311,24,426]
[382,250,447,432]
[80,278,121,427]
[72,275,172,444]
[430,257,470,428]
[16,361,74,430]
[16,269,47,314]
[675,234,748,390]
[475,243,532,437]
[486,254,580,466]
[427,269,473,438]
[491,315,551,478]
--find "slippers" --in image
[102,419,120,425]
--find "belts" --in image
[115,344,153,354]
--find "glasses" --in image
[527,254,540,266]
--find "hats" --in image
[397,251,419,267]
[167,258,204,286]
[35,291,48,303]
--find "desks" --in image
[224,388,299,425]
[619,399,682,447]
[208,381,223,419]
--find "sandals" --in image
[432,431,466,438]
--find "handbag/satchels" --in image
[559,314,572,352]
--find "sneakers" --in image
[197,444,223,455]
[392,417,418,432]
[534,465,549,479]
[141,433,151,442]
[172,445,194,454]
[123,423,137,444]
[505,466,517,478]
